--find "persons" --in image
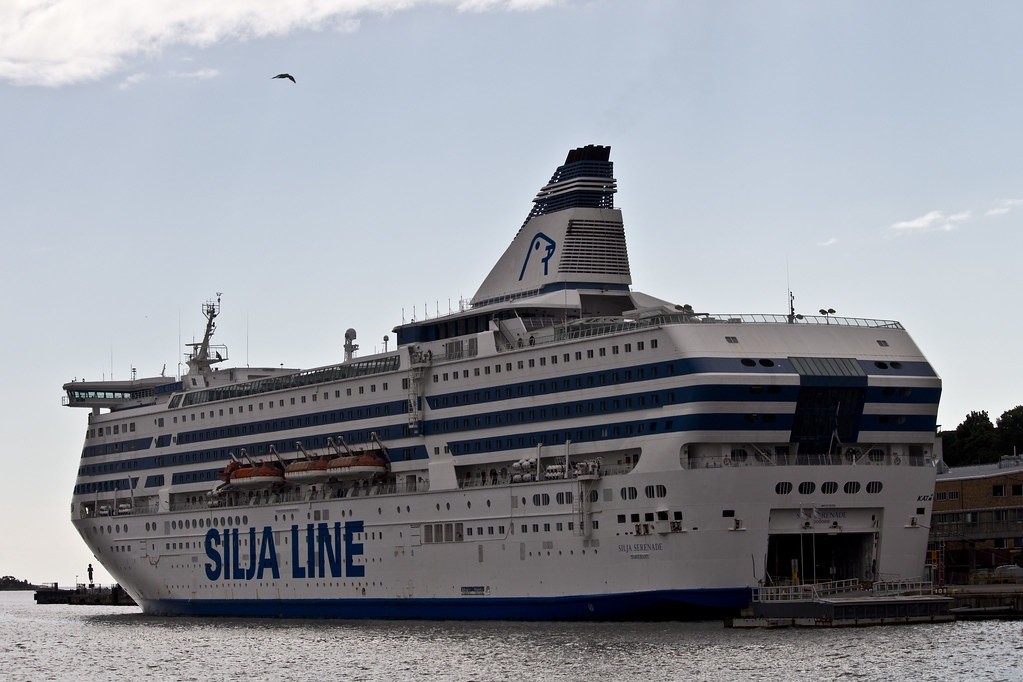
[311,486,316,499]
[354,480,359,496]
[724,455,732,467]
[490,467,497,485]
[363,479,369,490]
[372,478,378,487]
[481,469,486,486]
[894,456,901,465]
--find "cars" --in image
[967,568,994,585]
[994,564,1023,584]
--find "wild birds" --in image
[271,73,296,83]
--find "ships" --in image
[60,144,948,627]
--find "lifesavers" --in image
[724,458,731,465]
[894,457,901,465]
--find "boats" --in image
[229,465,284,490]
[326,454,388,484]
[283,458,329,485]
[722,516,959,630]
